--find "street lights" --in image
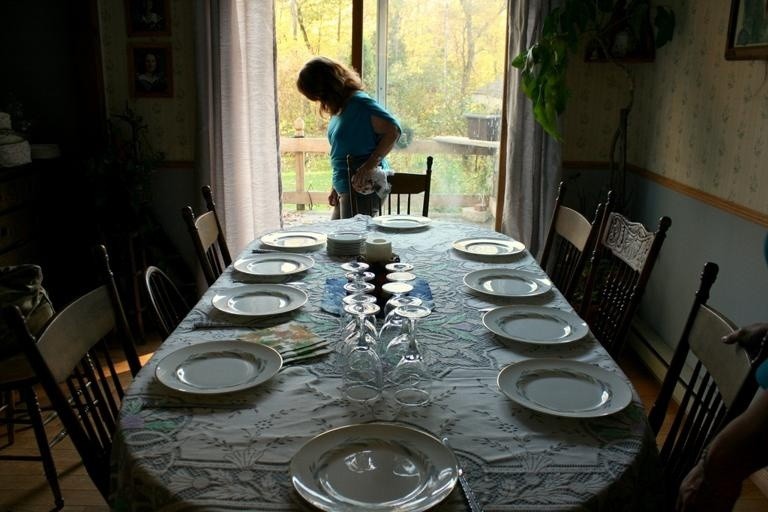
[0,262,57,359]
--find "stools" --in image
[0,337,94,506]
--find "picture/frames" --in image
[724,0,768,60]
[127,42,175,98]
[124,0,172,36]
[583,0,656,64]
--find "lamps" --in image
[347,153,433,217]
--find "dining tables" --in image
[510,0,676,146]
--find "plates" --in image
[373,215,432,230]
[157,339,284,396]
[211,283,309,317]
[288,423,459,510]
[497,357,632,419]
[234,251,313,277]
[481,304,590,346]
[452,235,527,257]
[259,230,327,249]
[463,267,553,298]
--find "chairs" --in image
[541,181,606,303]
[577,191,672,357]
[141,266,189,341]
[183,184,230,287]
[648,261,768,512]
[13,244,143,512]
[347,153,433,217]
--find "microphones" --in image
[0,337,94,506]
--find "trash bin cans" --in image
[373,153,384,163]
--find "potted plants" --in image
[124,0,172,36]
[583,0,656,64]
[510,0,676,146]
[127,42,175,98]
[724,0,768,60]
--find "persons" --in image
[136,50,163,92]
[296,56,401,220]
[674,320,768,512]
[134,1,162,34]
